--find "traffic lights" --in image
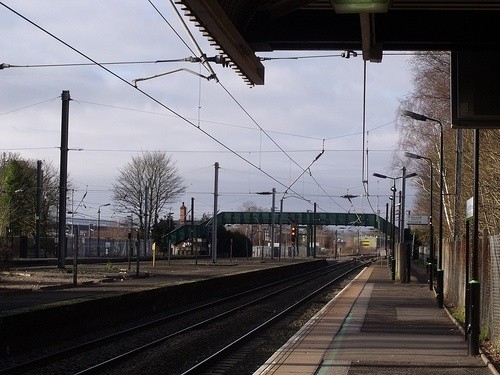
[292,226,296,238]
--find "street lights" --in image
[372,110,445,308]
[97,202,111,260]
